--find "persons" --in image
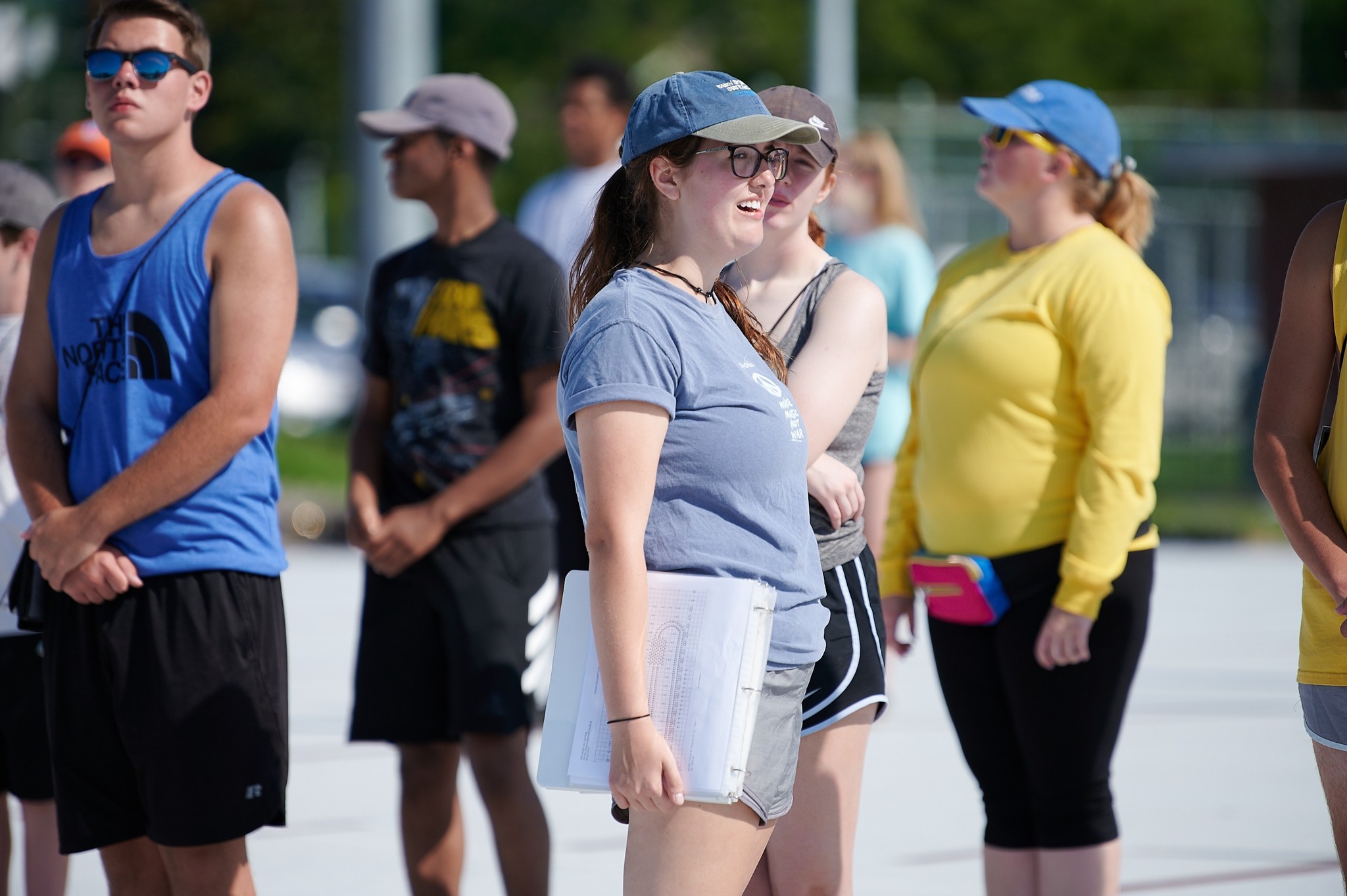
[346,74,591,896]
[1247,199,1347,896]
[516,60,637,635]
[558,70,832,896]
[51,118,117,207]
[876,78,1175,896]
[0,156,73,896]
[4,0,299,896]
[718,84,891,896]
[822,131,939,558]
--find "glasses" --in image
[670,145,790,181]
[986,120,1079,179]
[82,48,198,82]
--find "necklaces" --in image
[640,262,714,304]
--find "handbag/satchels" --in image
[8,536,73,633]
[908,551,1008,626]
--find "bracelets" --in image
[607,714,651,724]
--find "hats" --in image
[0,158,58,234]
[959,79,1122,179]
[621,71,821,167]
[356,73,518,160]
[757,86,840,168]
[56,117,111,166]
